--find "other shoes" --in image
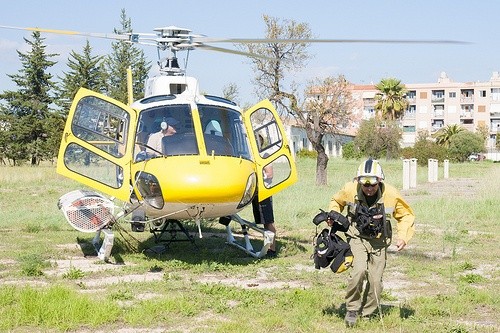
[266,250,277,256]
[344,311,358,327]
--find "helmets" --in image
[356,159,385,180]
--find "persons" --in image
[327,160,415,323]
[146,117,180,158]
[250,134,277,253]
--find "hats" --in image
[164,117,180,126]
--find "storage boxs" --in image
[58,189,114,233]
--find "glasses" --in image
[358,177,379,184]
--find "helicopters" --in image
[0,25,475,262]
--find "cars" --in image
[467,152,486,162]
